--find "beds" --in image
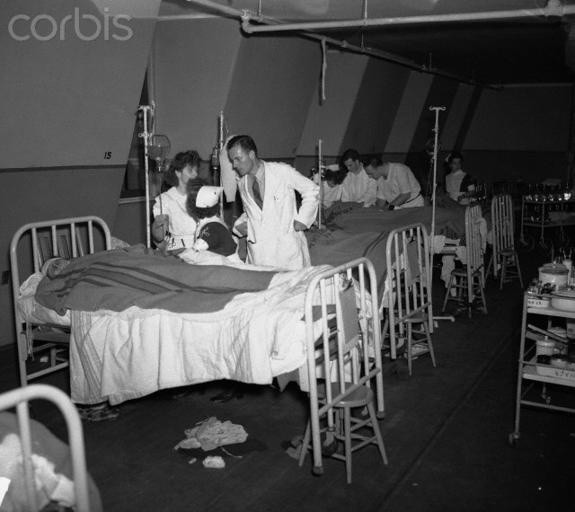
[0,186,508,509]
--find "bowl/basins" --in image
[550,288,575,312]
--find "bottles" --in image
[528,279,551,301]
[536,334,556,357]
[525,183,573,221]
[150,133,170,172]
[211,146,221,170]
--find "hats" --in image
[325,163,339,172]
[195,184,224,208]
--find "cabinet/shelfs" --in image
[515,289,575,446]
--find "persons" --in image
[308,147,426,210]
[150,134,320,271]
[444,153,477,207]
[42,256,72,279]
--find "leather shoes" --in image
[210,388,244,403]
